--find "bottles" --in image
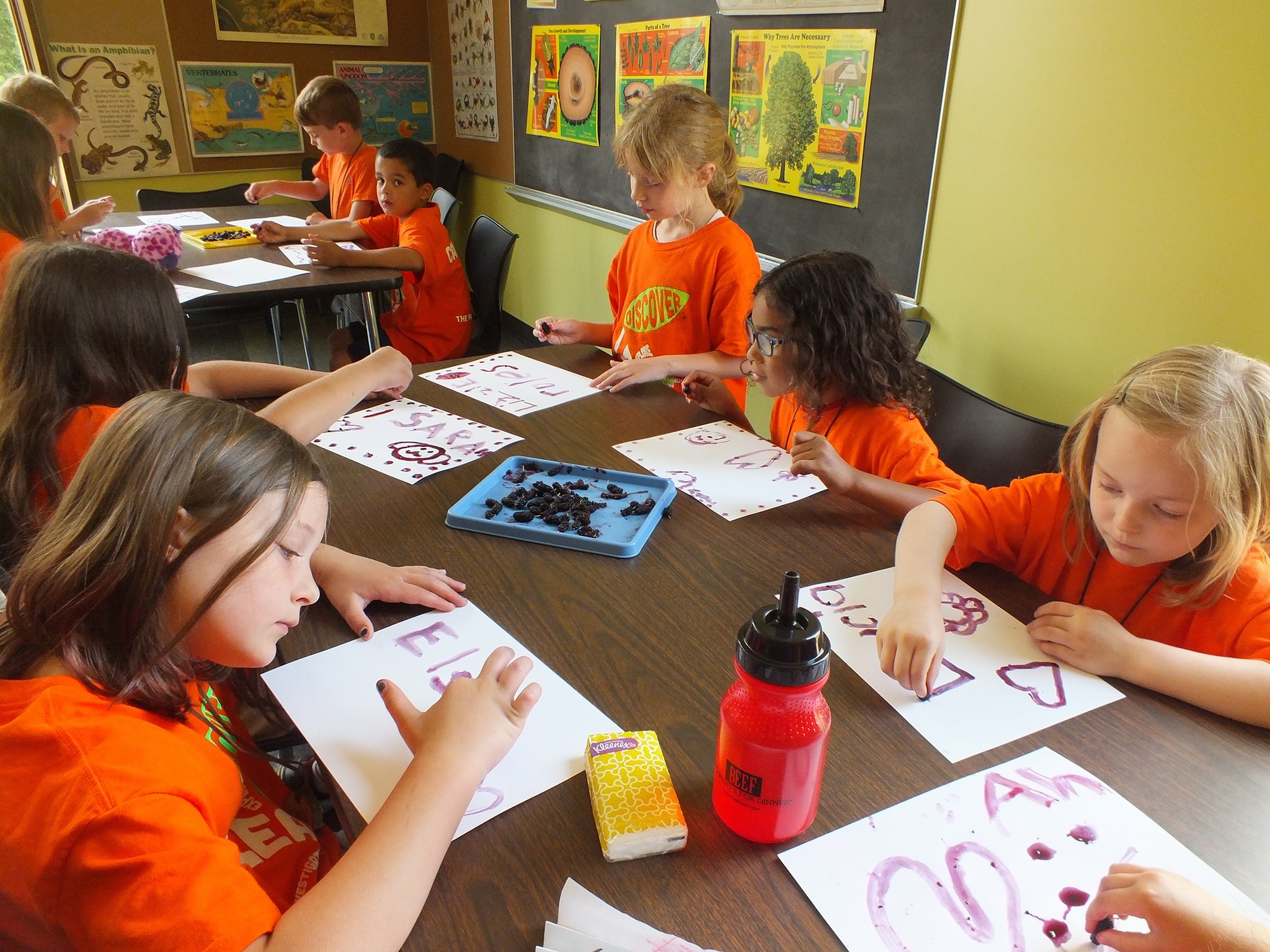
[712,571,833,845]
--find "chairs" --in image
[137,154,519,358]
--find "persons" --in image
[681,248,971,523]
[1085,862,1270,951]
[876,342,1270,728]
[532,80,762,413]
[0,69,117,310]
[244,76,385,328]
[250,137,473,373]
[0,232,541,952]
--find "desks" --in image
[203,340,1269,952]
[79,198,404,372]
[910,360,1071,491]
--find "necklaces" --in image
[785,396,849,451]
[332,139,364,219]
[1078,548,1165,626]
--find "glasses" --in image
[744,304,797,357]
